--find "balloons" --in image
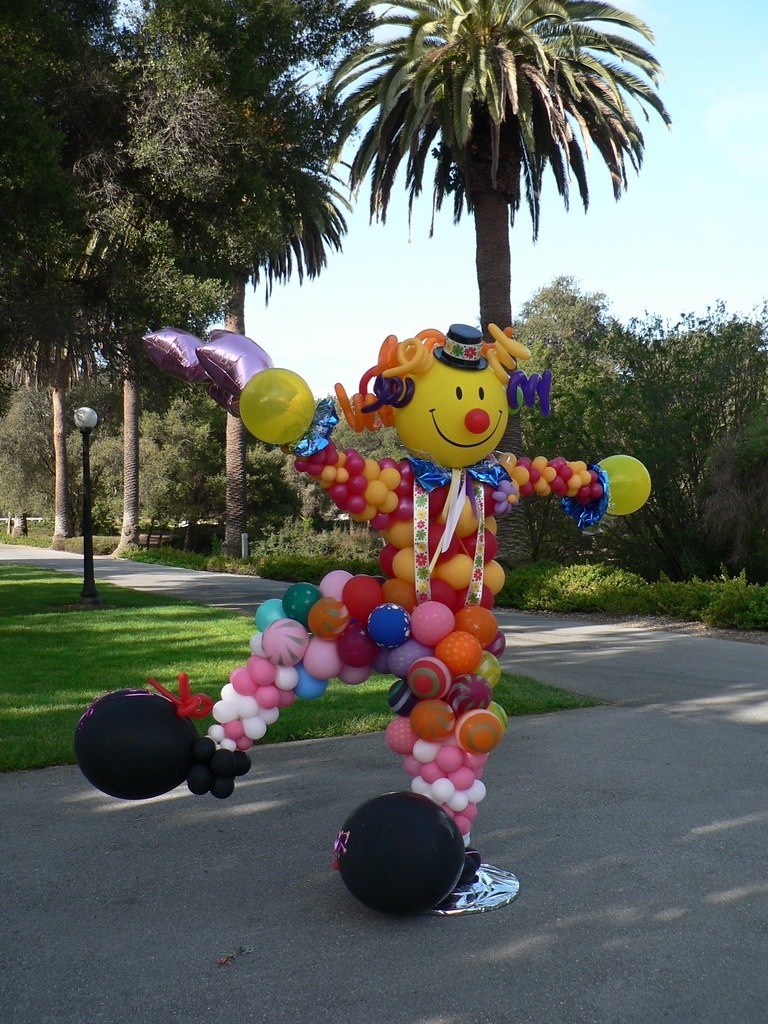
[337,791,467,917]
[76,689,199,801]
[142,324,652,886]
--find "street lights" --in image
[74,406,103,611]
